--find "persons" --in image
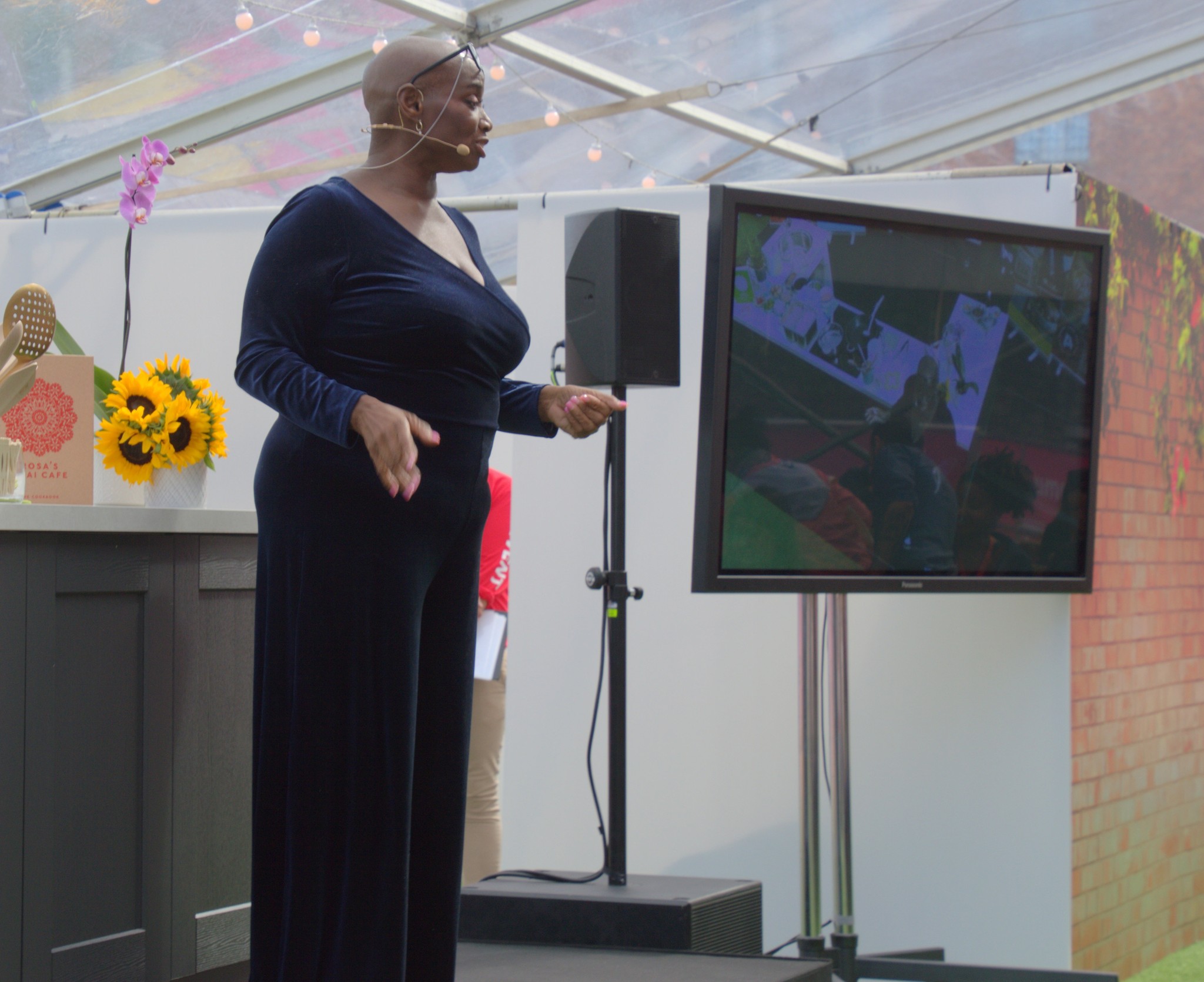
[230,30,635,982]
[725,392,1088,578]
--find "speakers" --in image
[567,209,683,388]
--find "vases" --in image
[142,453,209,509]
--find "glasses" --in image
[407,42,484,85]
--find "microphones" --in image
[372,123,469,156]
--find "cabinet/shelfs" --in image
[0,498,261,982]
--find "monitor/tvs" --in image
[692,185,1114,592]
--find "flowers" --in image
[95,350,230,490]
[106,134,197,377]
